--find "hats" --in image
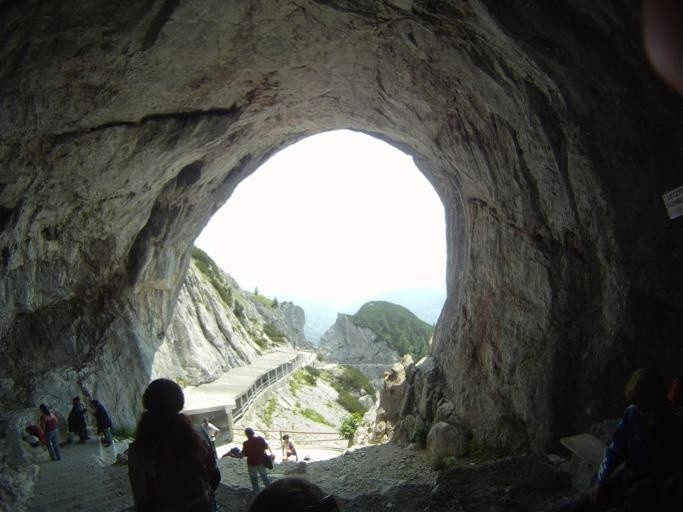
[142,379,184,412]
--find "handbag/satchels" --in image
[264,455,274,470]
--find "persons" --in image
[39,403,61,460]
[246,477,339,512]
[127,377,221,511]
[68,396,87,444]
[89,399,112,447]
[241,427,272,492]
[200,417,220,459]
[589,400,683,511]
[23,435,41,447]
[24,425,47,451]
[281,434,298,461]
